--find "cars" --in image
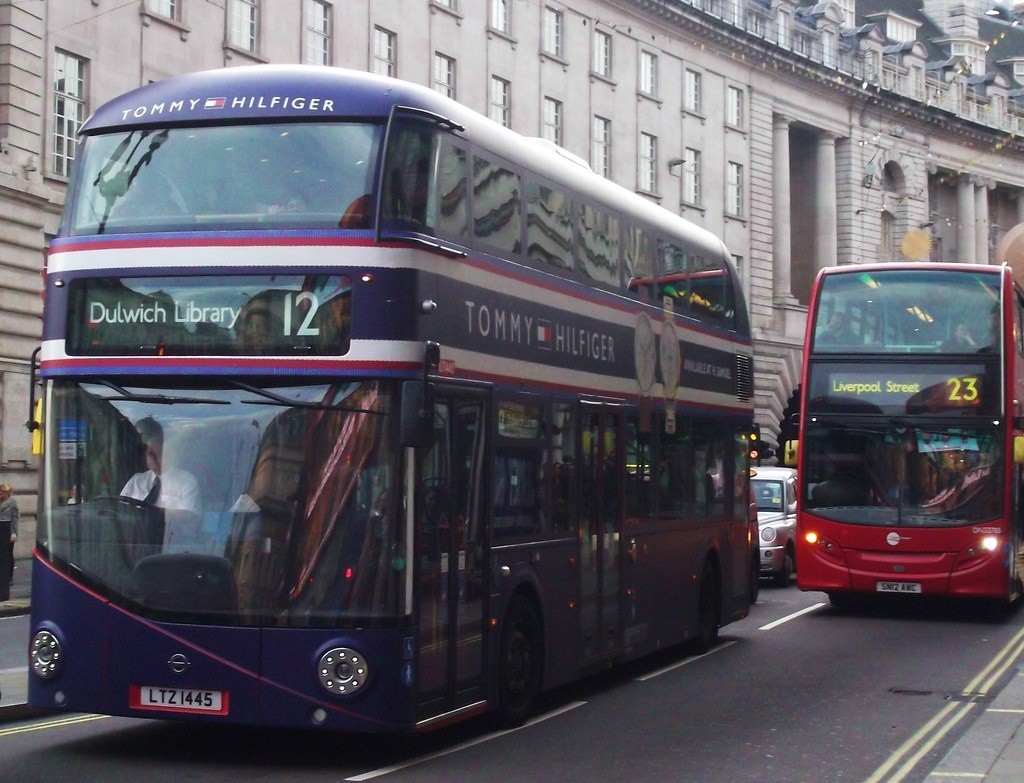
[747,465,799,587]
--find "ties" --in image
[143,476,161,504]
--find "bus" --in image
[26,61,761,739]
[784,261,1024,616]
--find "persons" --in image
[940,323,976,349]
[118,434,204,516]
[814,311,858,345]
[0,484,19,601]
